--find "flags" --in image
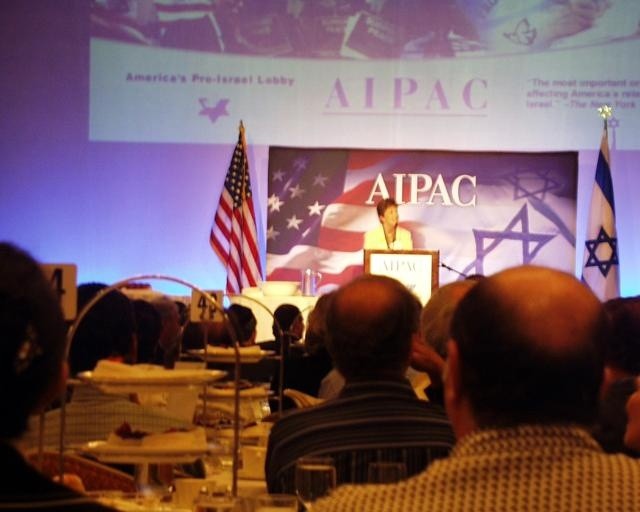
[264,144,577,295]
[209,126,262,300]
[581,126,621,303]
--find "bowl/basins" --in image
[254,280,303,296]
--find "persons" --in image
[311,265,640,512]
[586,294,640,457]
[420,279,480,360]
[264,275,457,512]
[0,241,112,512]
[68,283,335,414]
[363,198,414,251]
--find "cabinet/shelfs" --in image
[37,271,287,499]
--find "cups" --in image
[299,268,322,297]
